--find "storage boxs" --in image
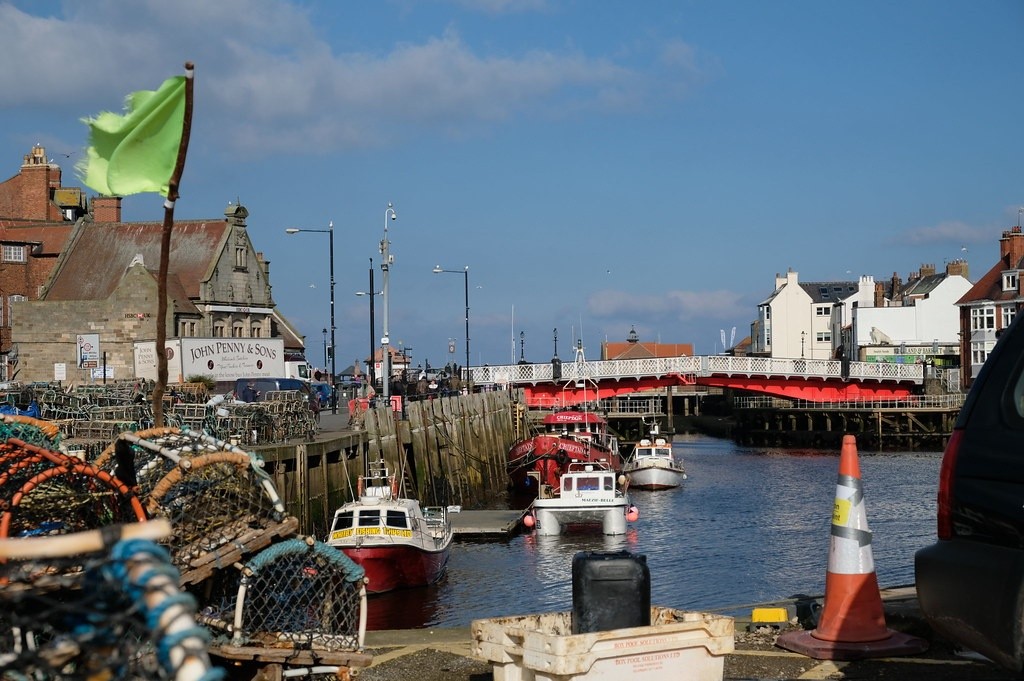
[470,605,735,681]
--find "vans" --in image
[234,378,321,425]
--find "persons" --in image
[243,382,261,403]
[357,375,461,403]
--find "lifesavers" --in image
[390,475,397,500]
[358,476,365,498]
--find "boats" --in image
[324,453,455,594]
[506,313,687,535]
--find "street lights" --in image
[379,203,397,406]
[519,331,527,362]
[801,331,807,358]
[433,265,472,395]
[552,327,559,359]
[355,257,383,407]
[286,222,339,414]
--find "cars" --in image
[310,382,339,410]
[914,305,1024,674]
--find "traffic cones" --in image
[775,435,929,659]
